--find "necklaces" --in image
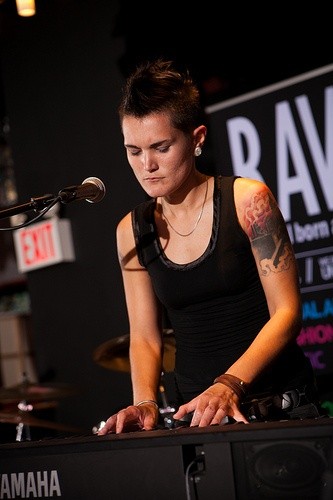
[160,175,209,236]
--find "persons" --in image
[98,61,324,437]
[0,114,38,388]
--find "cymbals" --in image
[1,412,81,434]
[93,328,177,374]
[1,380,90,410]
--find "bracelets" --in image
[213,374,249,401]
[136,400,158,410]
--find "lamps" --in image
[9,203,76,274]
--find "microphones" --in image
[59,177,106,205]
